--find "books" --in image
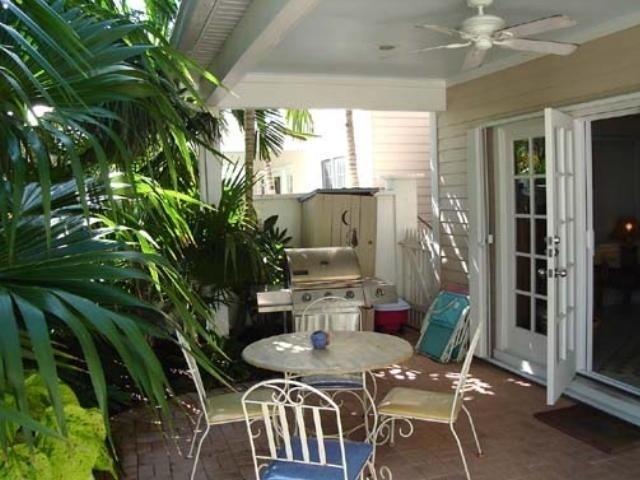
[374,297,411,335]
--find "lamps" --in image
[174,323,282,480]
[293,296,370,444]
[241,379,375,480]
[415,289,470,364]
[368,321,484,480]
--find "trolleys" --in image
[407,0,581,72]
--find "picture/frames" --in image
[241,330,414,477]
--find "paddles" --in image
[533,403,640,454]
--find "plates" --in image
[301,193,378,277]
